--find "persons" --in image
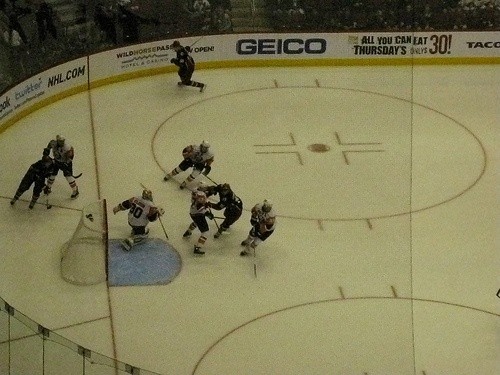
[9,151,64,210]
[112,188,166,250]
[164,139,216,191]
[239,198,278,257]
[182,180,214,257]
[174,1,500,39]
[207,182,243,241]
[170,40,207,93]
[0,0,162,96]
[44,134,81,198]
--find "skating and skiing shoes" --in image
[9,83,253,256]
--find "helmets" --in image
[42,40,273,213]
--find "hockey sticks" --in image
[46,186,52,209]
[140,183,168,239]
[206,201,222,235]
[49,157,83,179]
[254,218,258,278]
